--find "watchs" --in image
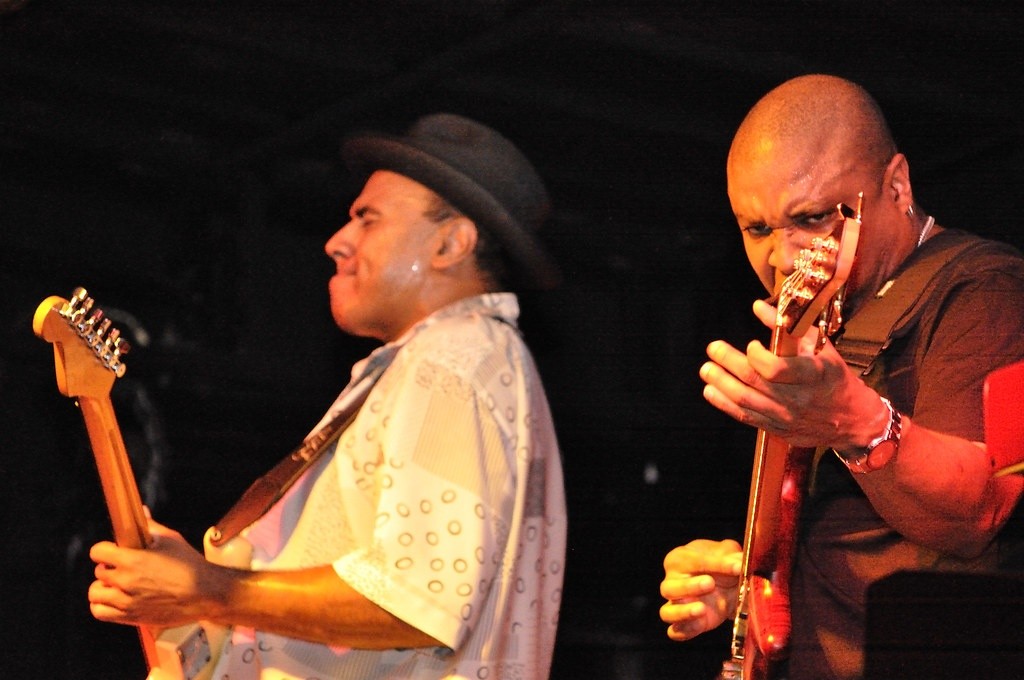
[833,396,902,474]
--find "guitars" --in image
[720,187,871,680]
[29,283,266,680]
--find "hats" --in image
[339,113,569,294]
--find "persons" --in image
[659,75,1024,680]
[89,113,568,680]
